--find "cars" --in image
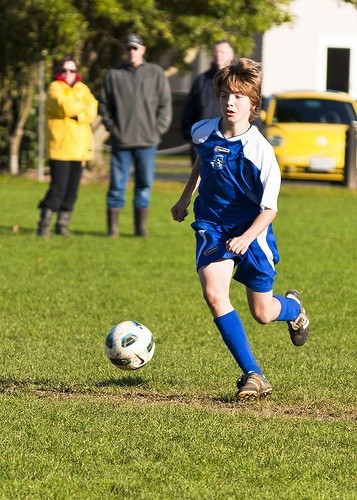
[253,87,357,183]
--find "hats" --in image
[124,33,145,46]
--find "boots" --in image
[106,207,120,237]
[133,207,148,237]
[56,209,71,235]
[39,207,53,238]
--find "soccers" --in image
[106,320,155,370]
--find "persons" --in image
[170,58,310,401]
[98,32,174,239]
[181,39,262,170]
[38,58,98,238]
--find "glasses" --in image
[126,45,143,51]
[61,69,77,74]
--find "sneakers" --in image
[237,374,271,403]
[285,289,308,347]
